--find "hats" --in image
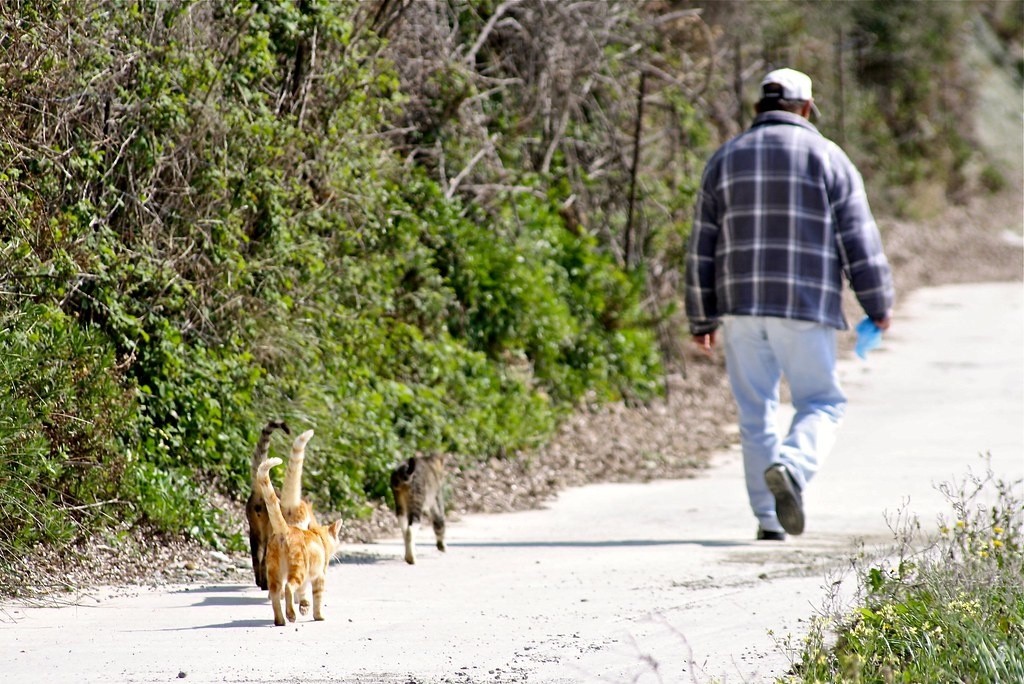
[760,68,821,118]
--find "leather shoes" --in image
[755,466,805,540]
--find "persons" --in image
[685,67,896,540]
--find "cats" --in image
[245,421,344,625]
[390,453,451,565]
